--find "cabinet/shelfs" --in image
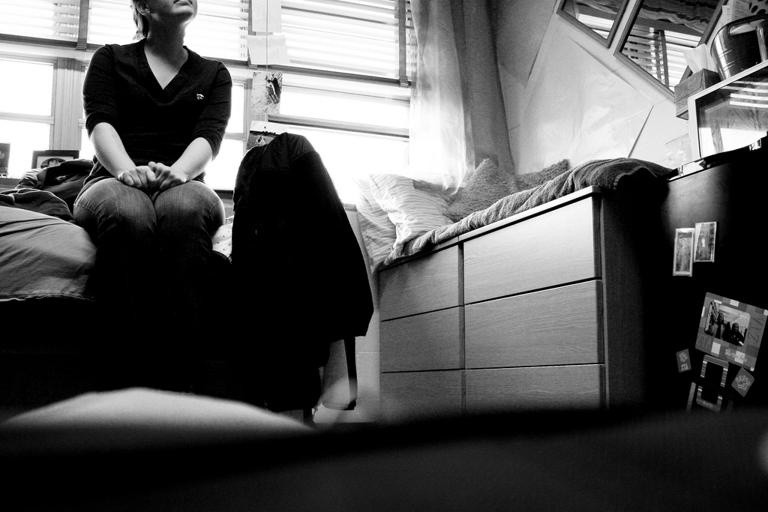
[375,185,663,423]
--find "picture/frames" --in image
[31,149,79,170]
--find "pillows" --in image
[356,157,569,275]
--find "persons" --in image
[266,74,281,103]
[72,0,232,264]
[704,302,747,346]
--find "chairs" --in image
[198,130,323,424]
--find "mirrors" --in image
[555,0,629,49]
[611,0,729,104]
